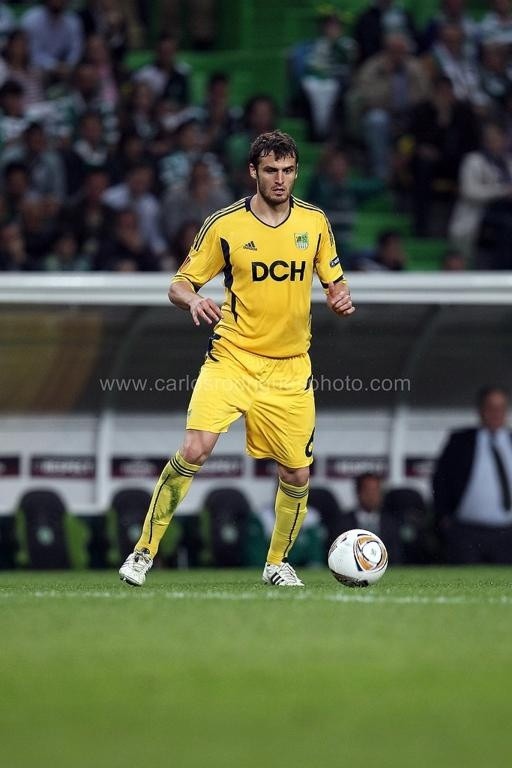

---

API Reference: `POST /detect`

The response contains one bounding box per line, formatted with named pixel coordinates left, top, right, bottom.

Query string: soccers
left=328, top=528, right=389, bottom=587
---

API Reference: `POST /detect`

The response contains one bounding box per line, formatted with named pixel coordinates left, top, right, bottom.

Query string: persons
left=335, top=469, right=401, bottom=556
left=119, top=127, right=354, bottom=588
left=430, top=384, right=510, bottom=568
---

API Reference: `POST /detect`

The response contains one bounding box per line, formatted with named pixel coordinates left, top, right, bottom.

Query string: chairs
left=1, top=484, right=430, bottom=572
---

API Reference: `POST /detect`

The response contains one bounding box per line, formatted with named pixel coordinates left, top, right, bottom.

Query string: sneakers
left=261, top=561, right=307, bottom=590
left=116, top=550, right=153, bottom=588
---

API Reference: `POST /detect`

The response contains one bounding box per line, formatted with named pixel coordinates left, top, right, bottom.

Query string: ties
left=488, top=440, right=510, bottom=511
